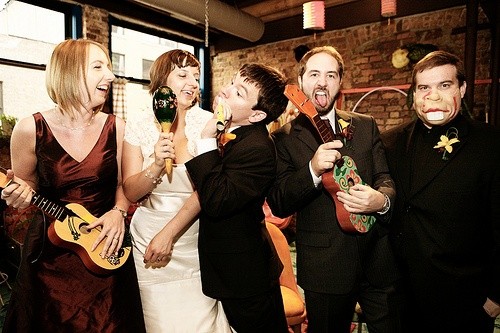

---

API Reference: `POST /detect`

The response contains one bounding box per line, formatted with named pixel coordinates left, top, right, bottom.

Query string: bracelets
left=145, top=167, right=161, bottom=184
left=112, top=206, right=128, bottom=219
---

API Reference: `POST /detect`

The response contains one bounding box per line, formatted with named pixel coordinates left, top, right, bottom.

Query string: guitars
left=285, top=84, right=377, bottom=234
left=0, top=167, right=132, bottom=273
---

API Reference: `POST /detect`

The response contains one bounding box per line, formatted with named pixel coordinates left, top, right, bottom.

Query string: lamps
left=303, top=1, right=325, bottom=40
left=380, top=0, right=397, bottom=25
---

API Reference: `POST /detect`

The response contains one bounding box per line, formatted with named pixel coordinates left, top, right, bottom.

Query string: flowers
left=337, top=119, right=351, bottom=146
left=433, top=127, right=460, bottom=161
left=218, top=132, right=236, bottom=157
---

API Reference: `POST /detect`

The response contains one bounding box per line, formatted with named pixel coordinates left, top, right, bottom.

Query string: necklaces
left=55, top=106, right=95, bottom=129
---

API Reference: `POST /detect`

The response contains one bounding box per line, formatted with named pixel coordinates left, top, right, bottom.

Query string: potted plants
left=0, top=114, right=18, bottom=136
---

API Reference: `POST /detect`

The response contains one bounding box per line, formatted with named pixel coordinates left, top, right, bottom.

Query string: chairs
left=265, top=221, right=307, bottom=333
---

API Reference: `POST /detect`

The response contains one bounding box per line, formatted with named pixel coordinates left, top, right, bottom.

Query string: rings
left=157, top=258, right=161, bottom=261
left=114, top=237, right=118, bottom=240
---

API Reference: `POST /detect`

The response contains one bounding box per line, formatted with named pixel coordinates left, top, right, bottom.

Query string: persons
left=121, top=49, right=237, bottom=333
left=381, top=50, right=500, bottom=333
left=265, top=46, right=397, bottom=333
left=184, top=64, right=289, bottom=333
left=1, top=39, right=133, bottom=333
